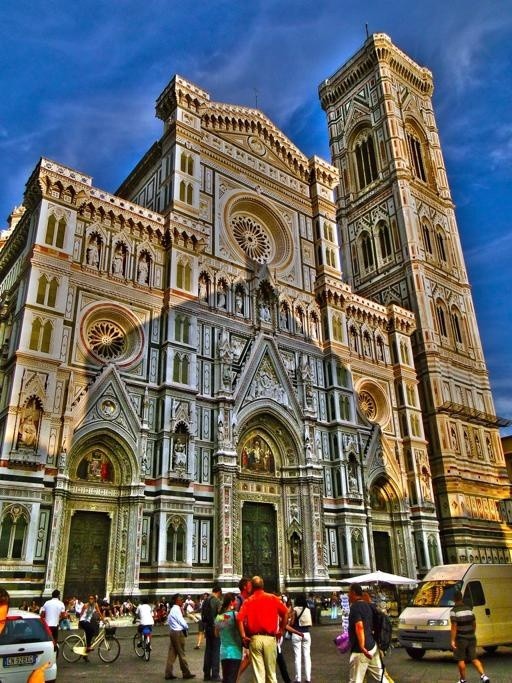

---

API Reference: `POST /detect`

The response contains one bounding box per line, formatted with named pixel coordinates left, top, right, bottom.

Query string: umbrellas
left=335, top=570, right=422, bottom=587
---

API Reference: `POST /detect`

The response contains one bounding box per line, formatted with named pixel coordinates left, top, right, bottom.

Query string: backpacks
left=371, top=605, right=391, bottom=650
left=202, top=596, right=214, bottom=623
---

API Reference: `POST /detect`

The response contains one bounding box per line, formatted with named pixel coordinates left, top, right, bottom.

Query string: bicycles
left=62, top=617, right=121, bottom=664
left=132, top=619, right=152, bottom=662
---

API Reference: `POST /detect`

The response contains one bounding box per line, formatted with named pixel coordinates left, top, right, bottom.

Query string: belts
left=252, top=632, right=275, bottom=637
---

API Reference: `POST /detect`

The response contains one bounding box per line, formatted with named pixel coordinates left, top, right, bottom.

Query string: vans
left=397, top=563, right=512, bottom=659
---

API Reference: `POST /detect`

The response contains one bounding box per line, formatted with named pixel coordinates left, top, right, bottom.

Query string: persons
left=249, top=367, right=289, bottom=407
left=241, top=441, right=276, bottom=473
left=172, top=438, right=187, bottom=466
left=447, top=592, right=490, bottom=682
left=258, top=295, right=383, bottom=361
left=235, top=290, right=244, bottom=313
left=216, top=284, right=226, bottom=308
left=1, top=577, right=395, bottom=682
left=137, top=256, right=148, bottom=284
left=113, top=249, right=124, bottom=278
left=198, top=277, right=208, bottom=301
left=87, top=240, right=100, bottom=266
left=77, top=455, right=115, bottom=481
left=18, top=414, right=36, bottom=446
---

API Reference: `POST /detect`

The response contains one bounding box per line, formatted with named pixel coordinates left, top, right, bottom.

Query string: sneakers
left=194, top=646, right=201, bottom=650
left=83, top=656, right=90, bottom=662
left=165, top=676, right=177, bottom=680
left=137, top=642, right=143, bottom=647
left=213, top=677, right=221, bottom=682
left=146, top=646, right=152, bottom=651
left=85, top=648, right=94, bottom=653
left=183, top=675, right=196, bottom=679
left=204, top=676, right=211, bottom=681
left=458, top=679, right=466, bottom=683
left=481, top=674, right=489, bottom=683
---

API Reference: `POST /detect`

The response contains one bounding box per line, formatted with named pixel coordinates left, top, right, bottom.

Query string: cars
left=0, top=609, right=59, bottom=683
